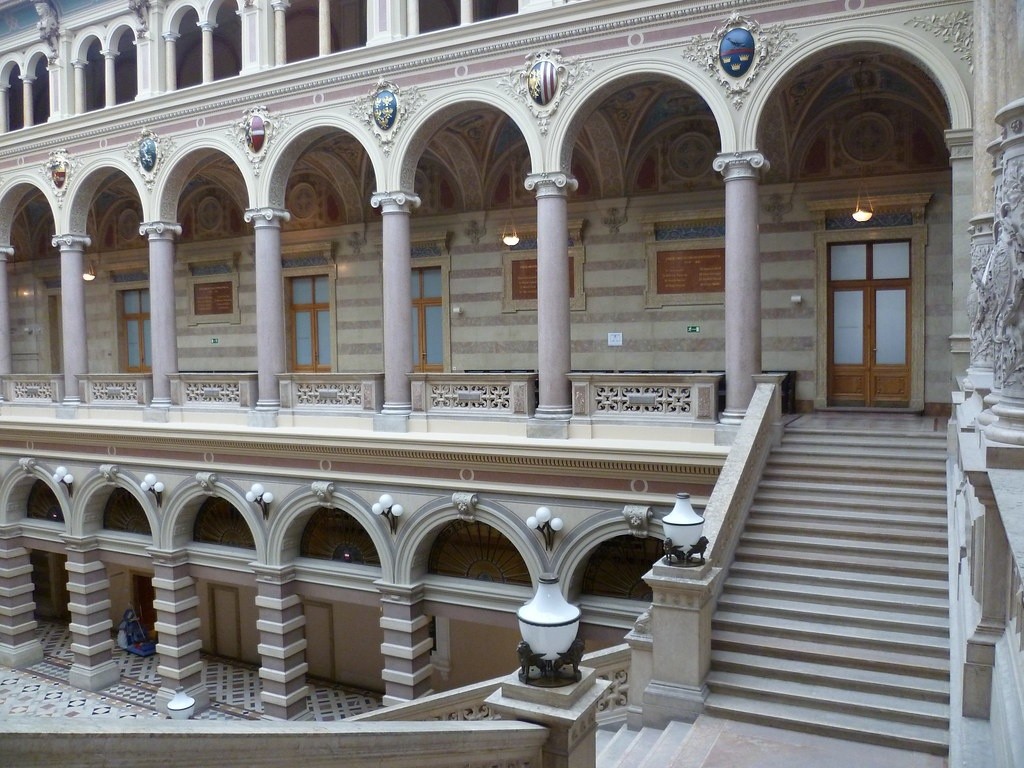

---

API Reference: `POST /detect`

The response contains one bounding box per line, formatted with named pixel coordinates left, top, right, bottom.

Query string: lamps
left=499, top=153, right=519, bottom=246
left=246, top=482, right=273, bottom=520
left=851, top=55, right=874, bottom=222
left=527, top=507, right=563, bottom=552
left=53, top=465, right=73, bottom=497
left=140, top=473, right=165, bottom=507
left=372, top=494, right=403, bottom=534
left=661, top=493, right=708, bottom=568
left=82, top=259, right=97, bottom=282
left=516, top=574, right=586, bottom=687
left=167, top=684, right=195, bottom=719
